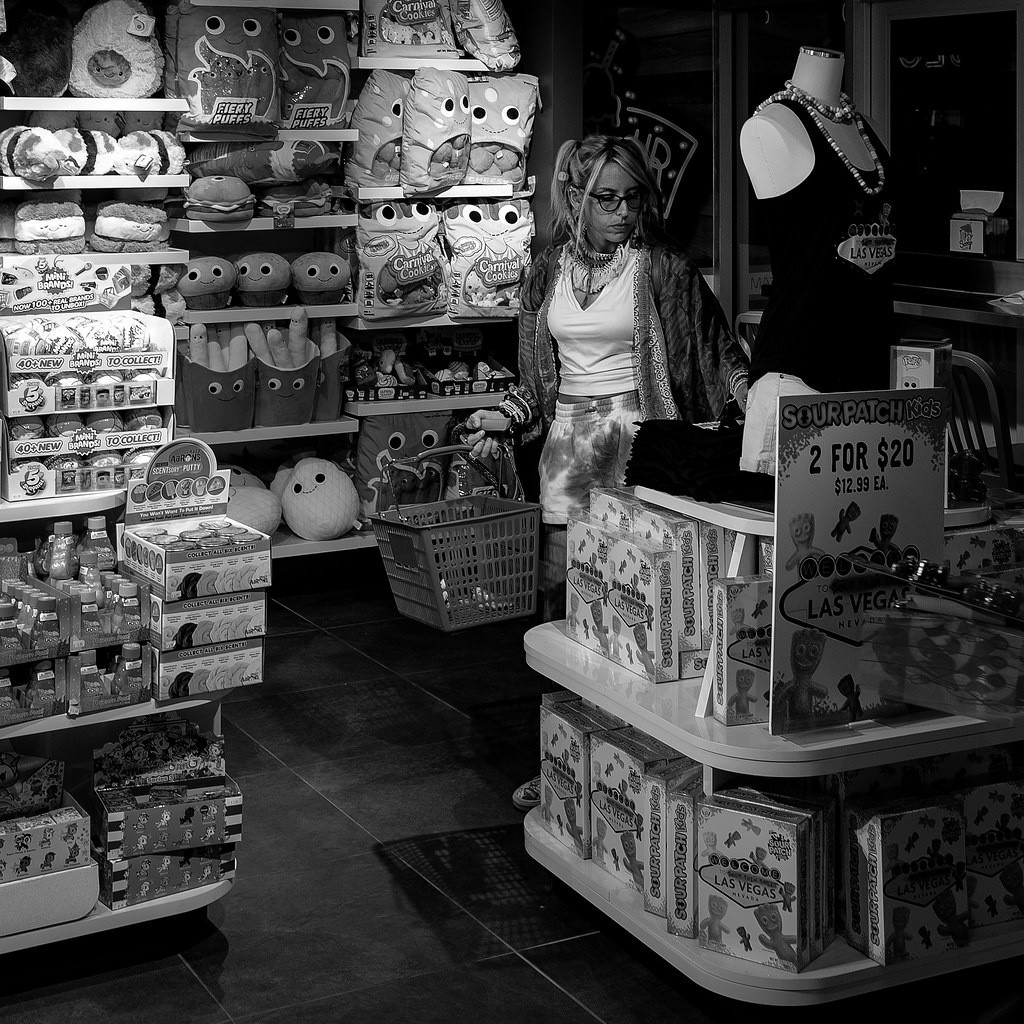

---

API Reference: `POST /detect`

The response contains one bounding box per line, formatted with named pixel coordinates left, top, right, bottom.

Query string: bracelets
left=492, top=382, right=539, bottom=432
left=726, top=367, right=749, bottom=404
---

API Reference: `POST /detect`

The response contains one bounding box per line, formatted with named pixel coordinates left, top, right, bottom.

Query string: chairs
left=946, top=350, right=1024, bottom=495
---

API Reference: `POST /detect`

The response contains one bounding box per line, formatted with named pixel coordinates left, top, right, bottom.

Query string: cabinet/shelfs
left=0, top=0, right=514, bottom=559
left=523, top=486, right=1024, bottom=1005
left=0, top=488, right=238, bottom=954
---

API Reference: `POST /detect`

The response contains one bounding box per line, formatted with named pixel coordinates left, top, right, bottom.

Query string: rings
left=469, top=452, right=478, bottom=460
left=465, top=436, right=469, bottom=445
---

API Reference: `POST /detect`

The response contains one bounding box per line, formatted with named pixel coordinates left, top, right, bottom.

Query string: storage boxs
left=541, top=486, right=1024, bottom=975
left=421, top=356, right=517, bottom=396
left=0, top=310, right=272, bottom=936
left=343, top=368, right=428, bottom=403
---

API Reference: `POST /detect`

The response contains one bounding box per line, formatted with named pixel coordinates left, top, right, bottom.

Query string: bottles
left=0, top=515, right=142, bottom=711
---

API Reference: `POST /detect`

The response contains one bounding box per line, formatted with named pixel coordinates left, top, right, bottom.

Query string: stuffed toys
left=351, top=0, right=540, bottom=517
left=132, top=8, right=362, bottom=546
left=0, top=0, right=185, bottom=255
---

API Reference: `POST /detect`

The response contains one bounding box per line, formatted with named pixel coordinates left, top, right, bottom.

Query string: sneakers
left=511, top=774, right=541, bottom=807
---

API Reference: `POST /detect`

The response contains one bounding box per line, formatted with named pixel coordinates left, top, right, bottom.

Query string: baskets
left=368, top=443, right=545, bottom=634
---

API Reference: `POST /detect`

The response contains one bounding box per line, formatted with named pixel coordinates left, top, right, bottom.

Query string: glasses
left=577, top=188, right=648, bottom=212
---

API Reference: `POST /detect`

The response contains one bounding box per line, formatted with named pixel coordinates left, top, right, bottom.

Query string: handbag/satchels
left=444, top=424, right=525, bottom=502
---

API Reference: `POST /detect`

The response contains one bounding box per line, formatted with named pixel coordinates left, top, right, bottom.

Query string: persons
left=465, top=135, right=747, bottom=811
left=739, top=46, right=900, bottom=503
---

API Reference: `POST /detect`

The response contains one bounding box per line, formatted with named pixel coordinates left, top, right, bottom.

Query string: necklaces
left=559, top=221, right=631, bottom=294
left=752, top=80, right=885, bottom=195
